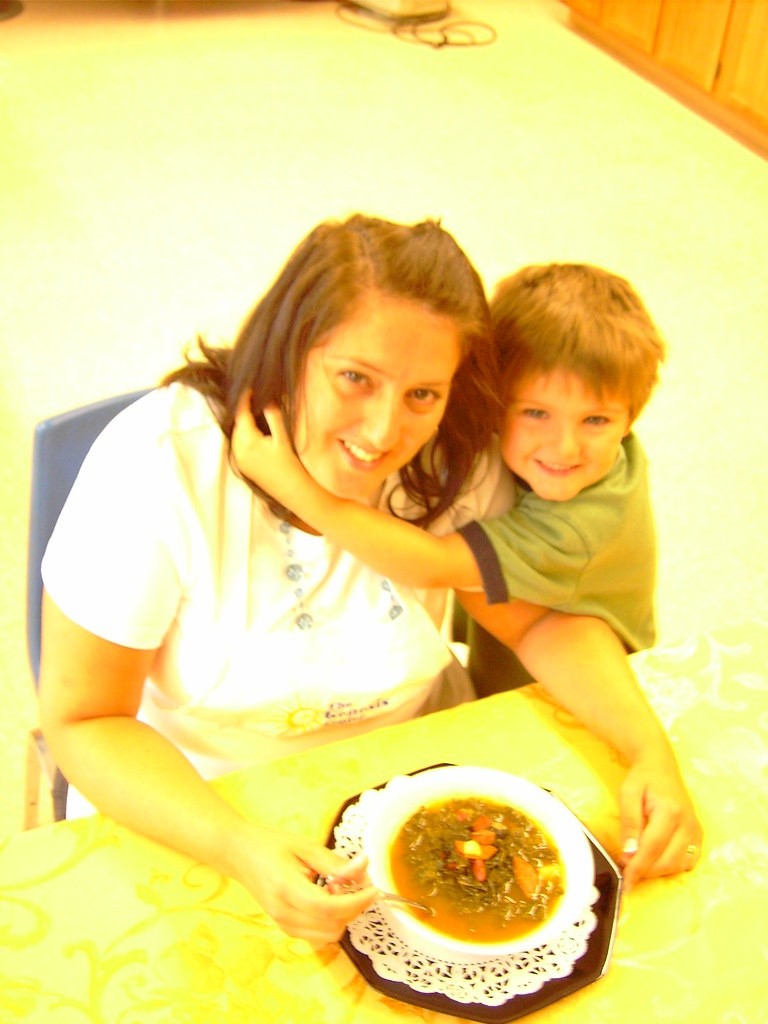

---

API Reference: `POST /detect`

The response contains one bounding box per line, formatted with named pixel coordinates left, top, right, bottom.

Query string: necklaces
left=281, top=508, right=405, bottom=634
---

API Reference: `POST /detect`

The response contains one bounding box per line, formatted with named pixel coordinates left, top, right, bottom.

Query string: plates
left=363, top=765, right=595, bottom=955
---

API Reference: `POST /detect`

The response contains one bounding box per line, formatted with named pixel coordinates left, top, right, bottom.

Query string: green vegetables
left=405, top=810, right=518, bottom=907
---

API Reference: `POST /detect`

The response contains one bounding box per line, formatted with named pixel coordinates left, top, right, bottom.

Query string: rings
left=685, top=845, right=696, bottom=856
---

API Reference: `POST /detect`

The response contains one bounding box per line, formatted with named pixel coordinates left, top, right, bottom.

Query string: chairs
left=22, top=387, right=158, bottom=832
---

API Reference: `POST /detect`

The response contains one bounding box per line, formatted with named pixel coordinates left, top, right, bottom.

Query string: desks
left=0, top=624, right=768, bottom=1022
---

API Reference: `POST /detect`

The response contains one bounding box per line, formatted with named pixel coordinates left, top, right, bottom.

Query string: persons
left=229, top=261, right=665, bottom=700
left=38, top=214, right=704, bottom=948
